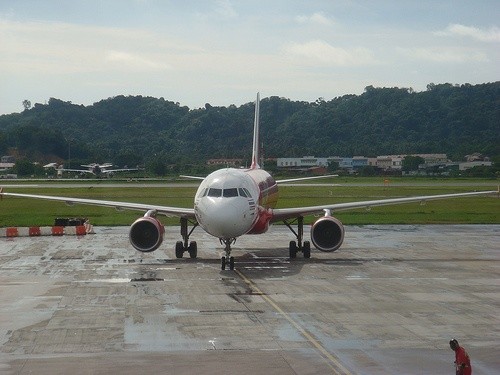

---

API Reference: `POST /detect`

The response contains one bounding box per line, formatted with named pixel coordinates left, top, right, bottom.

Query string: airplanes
left=0, top=92, right=499, bottom=272
left=55, top=161, right=146, bottom=179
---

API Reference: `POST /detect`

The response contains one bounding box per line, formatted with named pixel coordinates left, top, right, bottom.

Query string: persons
left=450, top=338, right=471, bottom=375
